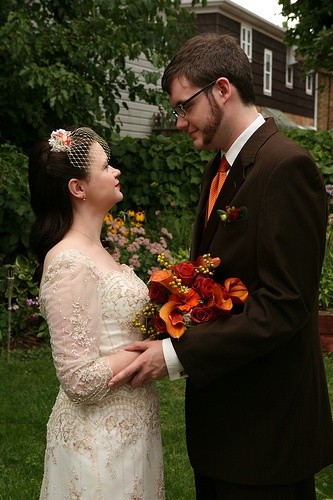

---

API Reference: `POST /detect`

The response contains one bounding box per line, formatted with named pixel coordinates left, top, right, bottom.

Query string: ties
left=208, top=154, right=229, bottom=221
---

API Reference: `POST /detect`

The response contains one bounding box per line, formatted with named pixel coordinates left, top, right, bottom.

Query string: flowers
left=128, top=253, right=248, bottom=339
left=216, top=204, right=239, bottom=221
left=48, top=128, right=74, bottom=152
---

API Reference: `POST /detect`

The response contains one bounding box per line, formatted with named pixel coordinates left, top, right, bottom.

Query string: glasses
left=172, top=81, right=215, bottom=118
